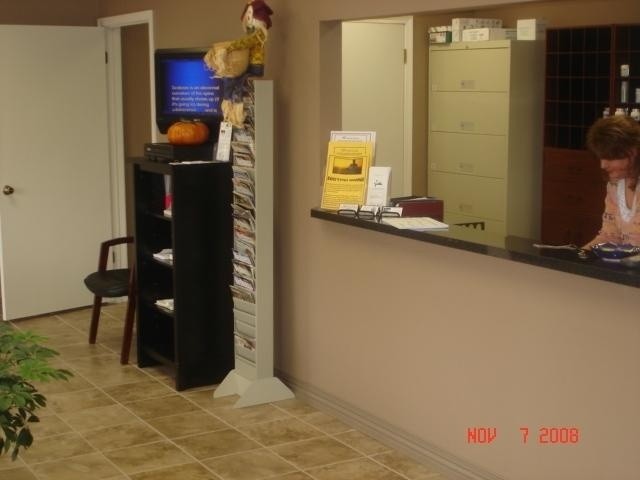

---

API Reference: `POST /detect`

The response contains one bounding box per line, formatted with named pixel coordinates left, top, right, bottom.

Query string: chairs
left=84, top=235, right=135, bottom=365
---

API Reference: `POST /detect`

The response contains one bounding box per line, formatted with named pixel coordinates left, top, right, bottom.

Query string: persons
left=577, top=116, right=640, bottom=262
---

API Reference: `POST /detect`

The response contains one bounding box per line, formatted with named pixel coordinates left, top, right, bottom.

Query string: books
left=215, top=115, right=255, bottom=355
left=319, top=128, right=394, bottom=211
left=382, top=215, right=450, bottom=233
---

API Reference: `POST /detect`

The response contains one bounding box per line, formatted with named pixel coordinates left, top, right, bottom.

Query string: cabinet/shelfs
left=615, top=22, right=640, bottom=119
left=132, top=154, right=234, bottom=391
left=543, top=24, right=616, bottom=245
left=425, top=42, right=546, bottom=247
left=212, top=80, right=293, bottom=405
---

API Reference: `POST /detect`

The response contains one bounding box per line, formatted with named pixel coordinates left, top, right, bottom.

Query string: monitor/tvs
left=154, top=48, right=224, bottom=143
